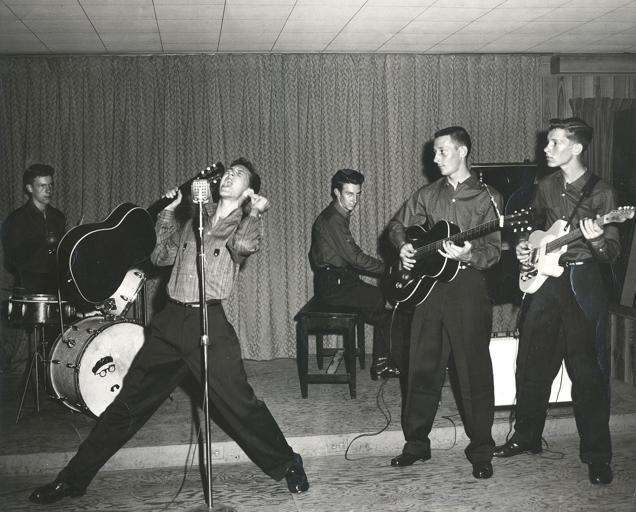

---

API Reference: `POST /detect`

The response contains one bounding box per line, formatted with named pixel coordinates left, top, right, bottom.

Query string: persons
left=309, top=168, right=402, bottom=381
left=387, top=125, right=503, bottom=480
left=492, top=117, right=622, bottom=483
left=1, top=163, right=67, bottom=338
left=29, top=157, right=309, bottom=506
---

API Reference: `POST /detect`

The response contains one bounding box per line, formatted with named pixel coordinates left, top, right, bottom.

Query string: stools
left=294, top=296, right=366, bottom=398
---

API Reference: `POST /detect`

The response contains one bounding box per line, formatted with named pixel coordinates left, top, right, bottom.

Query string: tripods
left=15, top=325, right=48, bottom=427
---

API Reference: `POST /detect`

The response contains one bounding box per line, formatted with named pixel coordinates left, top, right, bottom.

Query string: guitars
left=384, top=206, right=536, bottom=307
left=516, top=206, right=635, bottom=293
left=56, top=162, right=225, bottom=313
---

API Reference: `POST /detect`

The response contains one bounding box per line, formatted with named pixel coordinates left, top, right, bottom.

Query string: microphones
left=190, top=180, right=211, bottom=204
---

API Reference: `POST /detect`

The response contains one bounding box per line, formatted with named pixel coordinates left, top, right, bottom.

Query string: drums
left=7, top=293, right=76, bottom=329
left=47, top=315, right=146, bottom=419
left=94, top=269, right=148, bottom=316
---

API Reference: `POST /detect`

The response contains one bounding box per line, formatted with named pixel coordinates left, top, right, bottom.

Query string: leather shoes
left=491, top=440, right=542, bottom=458
left=286, top=465, right=308, bottom=493
left=588, top=463, right=612, bottom=484
left=473, top=464, right=492, bottom=479
left=370, top=365, right=400, bottom=381
left=390, top=452, right=430, bottom=468
left=28, top=480, right=85, bottom=505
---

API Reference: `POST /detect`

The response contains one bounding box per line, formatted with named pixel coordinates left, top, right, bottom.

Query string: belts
left=167, top=297, right=220, bottom=309
